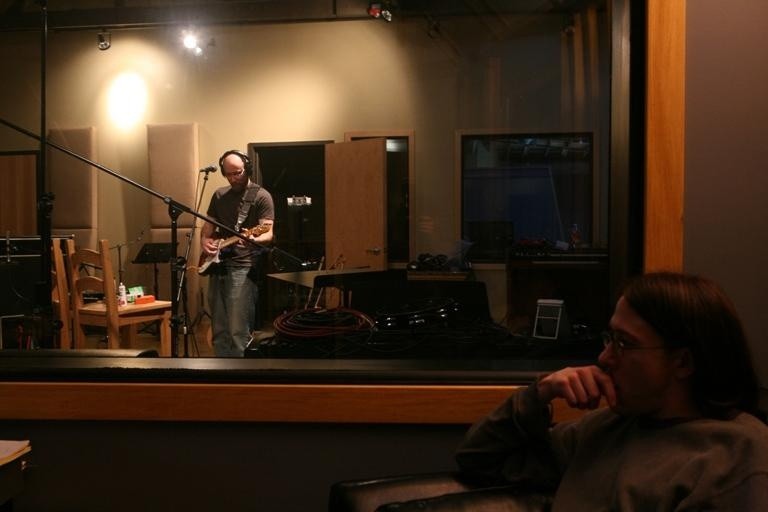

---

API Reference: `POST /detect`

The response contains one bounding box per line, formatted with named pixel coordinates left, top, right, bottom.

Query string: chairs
left=48, top=237, right=174, bottom=358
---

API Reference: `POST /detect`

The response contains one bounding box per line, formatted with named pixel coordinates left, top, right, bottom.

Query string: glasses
left=601, top=330, right=677, bottom=359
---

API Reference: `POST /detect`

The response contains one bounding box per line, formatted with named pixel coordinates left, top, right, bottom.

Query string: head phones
left=219, top=150, right=253, bottom=177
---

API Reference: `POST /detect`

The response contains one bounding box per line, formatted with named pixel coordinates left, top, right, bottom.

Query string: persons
left=198, top=150, right=276, bottom=358
left=455, top=270, right=766, bottom=509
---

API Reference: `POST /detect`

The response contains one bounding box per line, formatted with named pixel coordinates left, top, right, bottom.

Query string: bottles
left=118, top=282, right=128, bottom=307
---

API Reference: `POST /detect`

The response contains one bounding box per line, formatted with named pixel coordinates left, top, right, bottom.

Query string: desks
left=266, top=262, right=607, bottom=347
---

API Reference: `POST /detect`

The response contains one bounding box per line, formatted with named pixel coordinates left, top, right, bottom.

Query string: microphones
left=199, top=165, right=217, bottom=173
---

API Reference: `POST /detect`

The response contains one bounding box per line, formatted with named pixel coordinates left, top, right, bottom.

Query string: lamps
left=98, top=32, right=110, bottom=50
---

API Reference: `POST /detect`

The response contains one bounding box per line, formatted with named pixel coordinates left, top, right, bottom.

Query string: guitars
left=198, top=221, right=271, bottom=277
